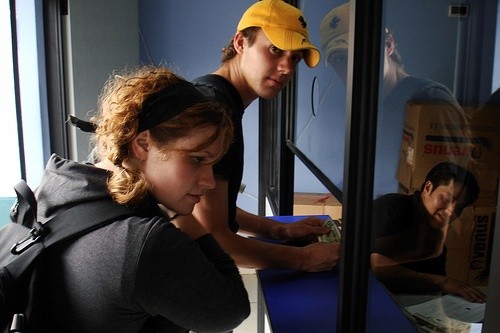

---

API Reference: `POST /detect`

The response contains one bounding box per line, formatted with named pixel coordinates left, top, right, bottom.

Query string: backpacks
left=2, top=180, right=170, bottom=333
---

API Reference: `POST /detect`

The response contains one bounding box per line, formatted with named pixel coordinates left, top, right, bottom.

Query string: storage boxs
left=395, top=99, right=500, bottom=287
left=293, top=192, right=342, bottom=220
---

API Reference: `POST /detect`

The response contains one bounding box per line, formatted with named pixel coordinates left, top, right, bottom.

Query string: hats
left=321, top=2, right=350, bottom=67
left=237, top=0, right=320, bottom=69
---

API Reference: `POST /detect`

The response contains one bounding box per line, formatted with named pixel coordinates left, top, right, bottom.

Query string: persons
left=13, top=67, right=251, bottom=333
left=189, top=0, right=340, bottom=273
left=369, top=161, right=488, bottom=303
left=321, top=2, right=464, bottom=194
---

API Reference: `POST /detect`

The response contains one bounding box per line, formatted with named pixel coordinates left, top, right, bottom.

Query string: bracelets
left=171, top=214, right=178, bottom=221
